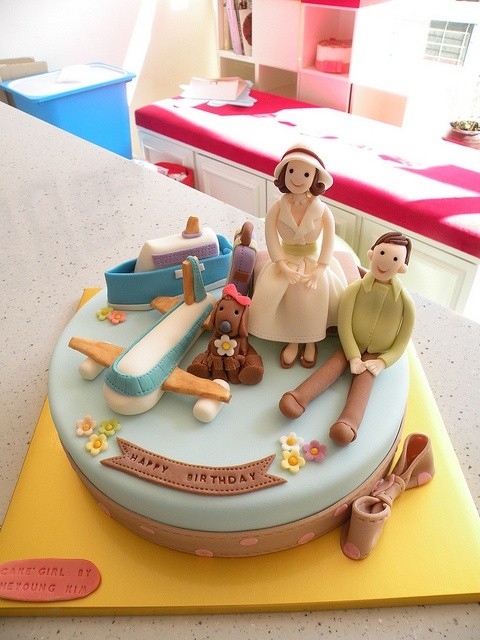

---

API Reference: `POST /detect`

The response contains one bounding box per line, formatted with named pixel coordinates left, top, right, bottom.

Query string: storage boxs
left=315, top=41, right=351, bottom=72
left=2, top=62, right=136, bottom=158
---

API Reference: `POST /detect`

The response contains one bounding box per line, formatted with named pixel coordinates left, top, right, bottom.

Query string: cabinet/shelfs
left=213, top=0, right=425, bottom=126
left=266, top=180, right=358, bottom=271
left=358, top=217, right=478, bottom=313
left=137, top=130, right=266, bottom=226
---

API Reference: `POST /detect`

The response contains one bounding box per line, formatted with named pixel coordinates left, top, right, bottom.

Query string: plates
left=451, top=127, right=480, bottom=136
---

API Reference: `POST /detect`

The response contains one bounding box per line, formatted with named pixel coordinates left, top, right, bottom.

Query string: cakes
left=45, top=141, right=436, bottom=561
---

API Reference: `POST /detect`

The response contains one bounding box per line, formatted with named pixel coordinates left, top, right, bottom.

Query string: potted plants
left=445, top=120, right=479, bottom=150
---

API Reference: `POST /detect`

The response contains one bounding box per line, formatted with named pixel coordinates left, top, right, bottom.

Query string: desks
left=0, top=102, right=480, bottom=639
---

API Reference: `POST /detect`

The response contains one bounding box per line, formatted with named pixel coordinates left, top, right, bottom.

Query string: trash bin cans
left=155, top=162, right=194, bottom=188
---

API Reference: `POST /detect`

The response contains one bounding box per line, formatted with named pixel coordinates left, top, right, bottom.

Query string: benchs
left=134, top=84, right=480, bottom=315
